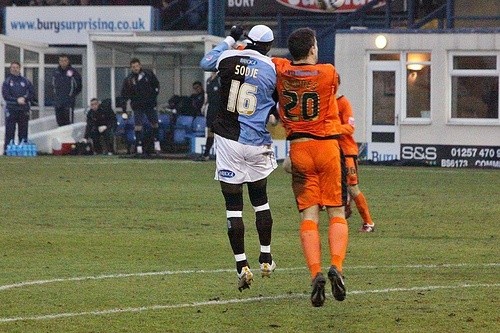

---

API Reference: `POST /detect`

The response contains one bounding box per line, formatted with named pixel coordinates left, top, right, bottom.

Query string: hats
left=242, top=25, right=274, bottom=43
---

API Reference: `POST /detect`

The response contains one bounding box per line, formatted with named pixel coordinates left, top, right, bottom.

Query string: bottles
left=6, top=144, right=36, bottom=157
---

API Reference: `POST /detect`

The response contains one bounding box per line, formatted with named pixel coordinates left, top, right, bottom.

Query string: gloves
left=230, top=23, right=244, bottom=40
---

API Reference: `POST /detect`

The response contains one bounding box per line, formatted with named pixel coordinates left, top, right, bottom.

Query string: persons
left=115, top=57, right=164, bottom=161
left=52, top=53, right=82, bottom=127
left=2, top=62, right=35, bottom=156
left=232, top=28, right=375, bottom=307
left=79, top=98, right=117, bottom=156
left=199, top=25, right=276, bottom=292
left=159, top=81, right=205, bottom=116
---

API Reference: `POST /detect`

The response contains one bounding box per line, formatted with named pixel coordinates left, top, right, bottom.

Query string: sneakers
left=327, top=265, right=346, bottom=301
left=236, top=266, right=254, bottom=293
left=259, top=260, right=276, bottom=278
left=310, top=273, right=326, bottom=307
left=358, top=222, right=375, bottom=234
left=344, top=205, right=353, bottom=219
left=194, top=154, right=209, bottom=162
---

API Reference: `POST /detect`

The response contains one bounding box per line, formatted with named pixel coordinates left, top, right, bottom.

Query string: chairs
left=112, top=111, right=206, bottom=155
